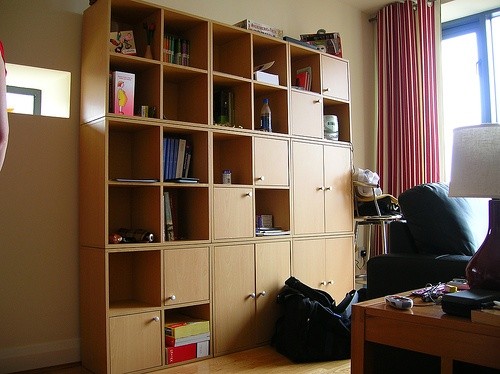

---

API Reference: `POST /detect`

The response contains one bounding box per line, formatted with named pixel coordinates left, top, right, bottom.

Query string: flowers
left=144, top=21, right=156, bottom=44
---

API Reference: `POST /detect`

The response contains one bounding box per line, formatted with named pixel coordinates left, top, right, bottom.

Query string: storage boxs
left=232, top=19, right=283, bottom=39
left=111, top=71, right=136, bottom=116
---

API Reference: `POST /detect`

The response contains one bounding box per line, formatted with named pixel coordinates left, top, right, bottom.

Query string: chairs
left=352, top=180, right=403, bottom=283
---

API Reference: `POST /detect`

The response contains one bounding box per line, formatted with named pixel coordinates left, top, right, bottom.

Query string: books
left=163, top=191, right=178, bottom=242
left=113, top=178, right=160, bottom=183
left=163, top=138, right=200, bottom=183
left=163, top=34, right=191, bottom=67
left=256, top=227, right=291, bottom=236
left=295, top=66, right=312, bottom=91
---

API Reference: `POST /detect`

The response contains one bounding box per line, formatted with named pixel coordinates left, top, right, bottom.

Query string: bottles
left=259, top=98, right=273, bottom=132
left=222, top=170, right=232, bottom=184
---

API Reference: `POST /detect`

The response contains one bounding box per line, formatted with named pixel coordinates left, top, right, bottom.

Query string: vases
left=144, top=45, right=154, bottom=59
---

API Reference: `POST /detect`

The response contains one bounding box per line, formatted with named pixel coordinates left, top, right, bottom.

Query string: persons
left=0, top=39, right=9, bottom=172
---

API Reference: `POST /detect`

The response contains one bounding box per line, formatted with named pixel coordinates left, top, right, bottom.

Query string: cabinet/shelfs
left=79, top=0, right=357, bottom=374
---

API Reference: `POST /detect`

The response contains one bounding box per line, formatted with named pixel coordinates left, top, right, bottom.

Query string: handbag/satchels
left=352, top=169, right=382, bottom=199
left=270, top=277, right=359, bottom=364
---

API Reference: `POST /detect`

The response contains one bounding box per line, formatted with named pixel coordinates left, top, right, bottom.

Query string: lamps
left=447, top=123, right=500, bottom=293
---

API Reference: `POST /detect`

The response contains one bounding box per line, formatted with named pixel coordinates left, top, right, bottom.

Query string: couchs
left=359, top=181, right=500, bottom=374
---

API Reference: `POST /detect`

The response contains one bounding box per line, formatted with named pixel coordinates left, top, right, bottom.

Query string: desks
left=351, top=288, right=500, bottom=374
